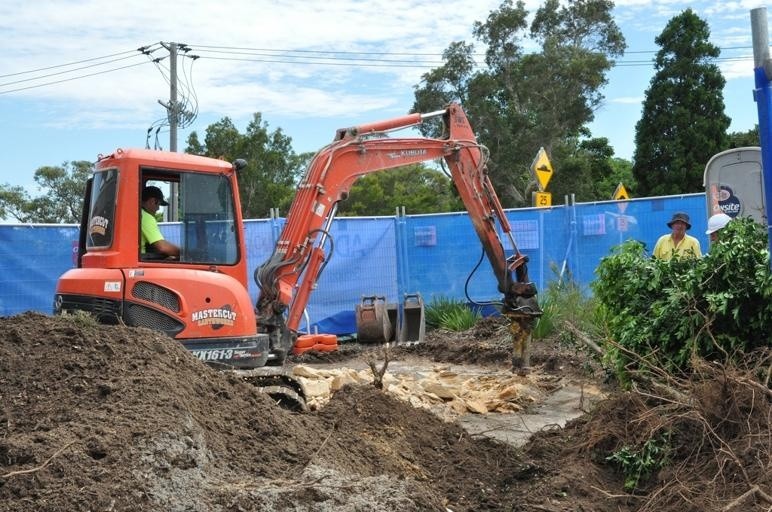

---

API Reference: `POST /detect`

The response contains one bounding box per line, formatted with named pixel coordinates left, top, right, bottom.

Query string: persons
left=652, top=213, right=702, bottom=267
left=705, top=214, right=732, bottom=243
left=138, top=185, right=181, bottom=260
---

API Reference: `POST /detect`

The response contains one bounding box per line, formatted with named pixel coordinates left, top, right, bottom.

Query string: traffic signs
left=534, top=190, right=552, bottom=208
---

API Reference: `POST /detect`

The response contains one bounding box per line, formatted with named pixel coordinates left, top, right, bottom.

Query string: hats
left=667, top=214, right=691, bottom=230
left=705, top=214, right=730, bottom=234
left=143, top=187, right=170, bottom=206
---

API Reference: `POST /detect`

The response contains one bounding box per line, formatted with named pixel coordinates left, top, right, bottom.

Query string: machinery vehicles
left=50, top=98, right=568, bottom=414
